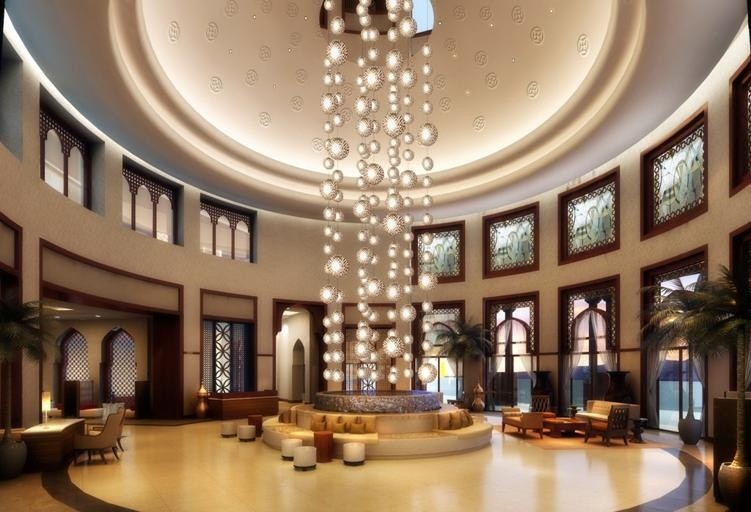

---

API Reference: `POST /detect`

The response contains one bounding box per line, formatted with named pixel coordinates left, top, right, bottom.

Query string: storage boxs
left=502, top=407, right=545, bottom=439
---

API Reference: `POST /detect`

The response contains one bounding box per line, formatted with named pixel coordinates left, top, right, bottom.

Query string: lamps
left=89, top=405, right=126, bottom=461
left=74, top=410, right=119, bottom=465
left=530, top=394, right=557, bottom=433
left=584, top=405, right=630, bottom=446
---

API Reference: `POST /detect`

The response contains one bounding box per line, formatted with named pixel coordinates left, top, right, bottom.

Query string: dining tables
left=434, top=315, right=496, bottom=407
left=634, top=274, right=705, bottom=445
left=1, top=295, right=59, bottom=479
left=642, top=263, right=751, bottom=512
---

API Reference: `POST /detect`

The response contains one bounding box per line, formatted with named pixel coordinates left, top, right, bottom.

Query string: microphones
left=629, top=417, right=648, bottom=444
left=221, top=413, right=369, bottom=470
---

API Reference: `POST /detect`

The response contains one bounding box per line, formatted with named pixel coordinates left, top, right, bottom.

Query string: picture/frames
left=21, top=417, right=85, bottom=465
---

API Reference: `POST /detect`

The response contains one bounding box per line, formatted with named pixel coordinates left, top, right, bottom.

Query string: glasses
left=320, top=1, right=439, bottom=389
left=42, top=391, right=52, bottom=428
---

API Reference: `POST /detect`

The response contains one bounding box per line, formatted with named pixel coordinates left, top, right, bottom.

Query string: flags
left=532, top=370, right=555, bottom=411
left=605, top=370, right=633, bottom=402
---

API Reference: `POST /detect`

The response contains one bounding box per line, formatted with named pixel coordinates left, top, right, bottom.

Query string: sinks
left=575, top=399, right=641, bottom=445
